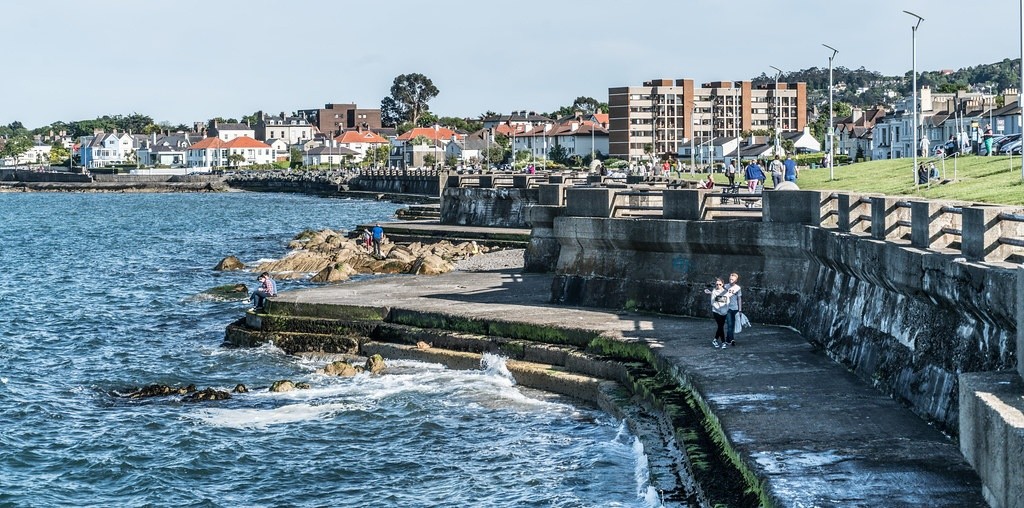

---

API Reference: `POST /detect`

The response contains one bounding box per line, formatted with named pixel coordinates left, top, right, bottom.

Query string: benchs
left=741, top=185, right=764, bottom=208
left=702, top=181, right=715, bottom=188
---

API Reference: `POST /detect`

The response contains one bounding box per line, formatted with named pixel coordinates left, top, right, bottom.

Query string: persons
left=362, top=228, right=372, bottom=245
left=700, top=174, right=712, bottom=189
left=783, top=157, right=798, bottom=183
left=820, top=152, right=830, bottom=168
left=242, top=272, right=277, bottom=313
left=963, top=124, right=993, bottom=156
left=599, top=163, right=607, bottom=182
left=704, top=273, right=742, bottom=345
left=711, top=277, right=730, bottom=349
left=920, top=135, right=930, bottom=157
left=524, top=164, right=534, bottom=174
left=769, top=155, right=783, bottom=187
left=629, top=158, right=685, bottom=178
left=745, top=159, right=767, bottom=193
left=728, top=160, right=736, bottom=185
left=918, top=161, right=938, bottom=183
left=371, top=222, right=383, bottom=254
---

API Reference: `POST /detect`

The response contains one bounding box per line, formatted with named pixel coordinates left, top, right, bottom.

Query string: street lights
left=822, top=43, right=839, bottom=182
left=903, top=9, right=925, bottom=186
left=985, top=83, right=995, bottom=133
left=368, top=132, right=396, bottom=170
left=133, top=136, right=143, bottom=163
left=731, top=80, right=742, bottom=178
left=426, top=123, right=441, bottom=170
left=708, top=93, right=717, bottom=174
left=769, top=65, right=783, bottom=154
left=689, top=101, right=697, bottom=173
left=482, top=123, right=547, bottom=175
left=587, top=120, right=596, bottom=161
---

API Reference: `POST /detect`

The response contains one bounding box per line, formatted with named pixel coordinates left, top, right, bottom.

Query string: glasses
left=716, top=284, right=722, bottom=286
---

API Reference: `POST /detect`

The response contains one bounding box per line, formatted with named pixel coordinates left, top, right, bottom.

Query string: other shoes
left=727, top=342, right=732, bottom=345
left=731, top=340, right=736, bottom=346
left=722, top=342, right=728, bottom=349
left=249, top=308, right=256, bottom=311
left=712, top=340, right=720, bottom=348
left=242, top=300, right=250, bottom=304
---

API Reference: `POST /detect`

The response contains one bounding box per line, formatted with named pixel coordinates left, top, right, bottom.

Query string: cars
left=963, top=133, right=1023, bottom=155
left=500, top=164, right=512, bottom=170
